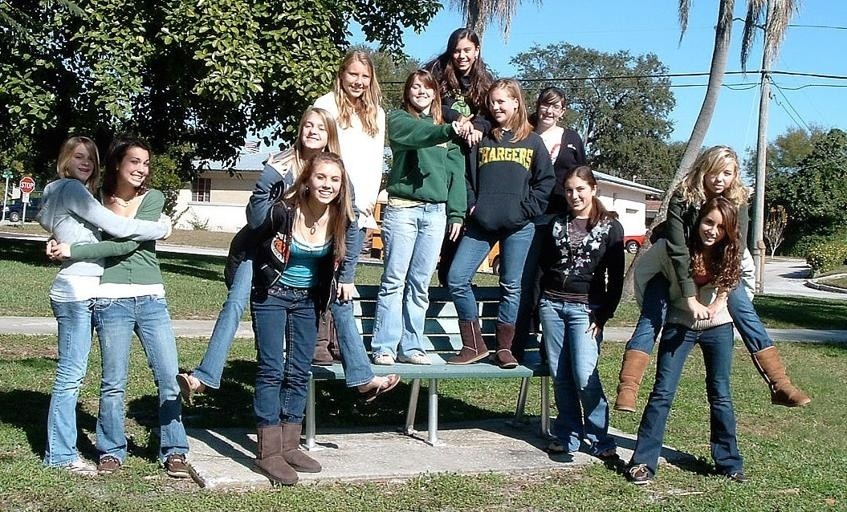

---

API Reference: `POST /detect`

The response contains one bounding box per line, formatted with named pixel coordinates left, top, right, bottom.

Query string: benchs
left=301, top=281, right=558, bottom=453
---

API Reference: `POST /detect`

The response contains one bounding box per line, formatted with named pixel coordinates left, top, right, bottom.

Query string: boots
left=282, top=422, right=322, bottom=473
left=313, top=310, right=333, bottom=366
left=447, top=319, right=489, bottom=365
left=251, top=425, right=299, bottom=485
left=494, top=323, right=518, bottom=368
left=614, top=349, right=650, bottom=413
left=751, top=346, right=812, bottom=407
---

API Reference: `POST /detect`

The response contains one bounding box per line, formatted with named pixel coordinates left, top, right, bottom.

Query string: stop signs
left=19, top=176, right=36, bottom=194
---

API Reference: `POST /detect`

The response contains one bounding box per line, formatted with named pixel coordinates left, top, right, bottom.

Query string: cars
left=623, top=230, right=648, bottom=253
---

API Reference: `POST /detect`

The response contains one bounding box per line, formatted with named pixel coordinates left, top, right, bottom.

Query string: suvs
left=0, top=197, right=42, bottom=222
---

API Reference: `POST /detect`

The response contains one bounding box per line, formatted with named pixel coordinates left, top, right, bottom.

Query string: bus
left=370, top=187, right=501, bottom=278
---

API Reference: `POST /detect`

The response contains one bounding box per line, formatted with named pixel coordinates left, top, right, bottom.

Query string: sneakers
left=549, top=440, right=579, bottom=451
left=731, top=473, right=747, bottom=482
left=629, top=463, right=649, bottom=485
left=167, top=454, right=190, bottom=478
left=62, top=458, right=98, bottom=476
left=397, top=353, right=432, bottom=365
left=98, top=456, right=121, bottom=475
left=373, top=353, right=395, bottom=365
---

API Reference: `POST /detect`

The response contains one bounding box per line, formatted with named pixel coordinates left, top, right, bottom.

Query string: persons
left=36, top=136, right=173, bottom=473
left=177, top=107, right=400, bottom=406
left=615, top=145, right=811, bottom=413
left=311, top=47, right=384, bottom=363
left=511, top=86, right=587, bottom=364
left=370, top=69, right=472, bottom=365
left=46, top=140, right=188, bottom=478
left=223, top=153, right=354, bottom=486
left=445, top=79, right=557, bottom=367
left=426, top=29, right=494, bottom=286
left=628, top=198, right=756, bottom=485
left=538, top=167, right=622, bottom=457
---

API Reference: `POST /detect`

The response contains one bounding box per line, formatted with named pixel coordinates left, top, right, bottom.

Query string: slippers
left=176, top=374, right=200, bottom=408
left=366, top=374, right=400, bottom=403
